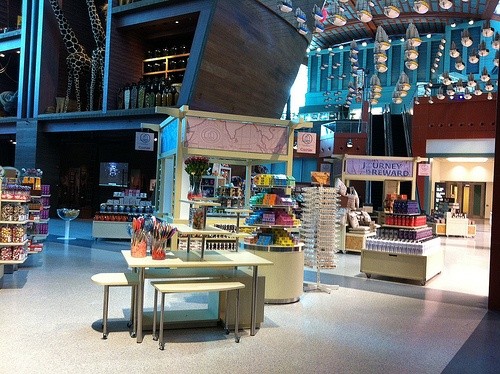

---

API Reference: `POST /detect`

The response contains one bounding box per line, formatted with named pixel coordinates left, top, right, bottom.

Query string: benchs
left=90, top=272, right=137, bottom=340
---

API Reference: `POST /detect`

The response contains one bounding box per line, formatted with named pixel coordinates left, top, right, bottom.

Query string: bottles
left=0, top=224, right=24, bottom=244
left=0, top=245, right=25, bottom=261
left=1, top=203, right=29, bottom=221
left=365, top=213, right=469, bottom=255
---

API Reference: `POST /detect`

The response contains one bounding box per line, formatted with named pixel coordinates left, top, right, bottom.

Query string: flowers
left=184, top=154, right=209, bottom=174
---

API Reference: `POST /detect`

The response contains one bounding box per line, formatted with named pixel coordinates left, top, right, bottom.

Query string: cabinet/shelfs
left=300, top=187, right=341, bottom=294
left=382, top=210, right=427, bottom=230
left=142, top=52, right=190, bottom=79
left=240, top=184, right=302, bottom=228
left=202, top=164, right=231, bottom=197
left=0, top=195, right=51, bottom=264
left=100, top=203, right=133, bottom=214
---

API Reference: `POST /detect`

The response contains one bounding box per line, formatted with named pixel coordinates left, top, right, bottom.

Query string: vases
left=187, top=175, right=203, bottom=200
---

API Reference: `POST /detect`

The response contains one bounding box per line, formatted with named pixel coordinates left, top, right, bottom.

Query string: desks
left=445, top=211, right=468, bottom=238
left=120, top=250, right=274, bottom=339
left=169, top=223, right=232, bottom=261
left=178, top=200, right=221, bottom=228
left=427, top=222, right=446, bottom=237
left=468, top=225, right=476, bottom=238
left=92, top=221, right=132, bottom=241
left=150, top=279, right=246, bottom=351
left=346, top=233, right=372, bottom=253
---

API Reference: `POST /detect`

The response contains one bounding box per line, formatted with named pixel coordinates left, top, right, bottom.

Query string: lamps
left=278, top=0, right=500, bottom=108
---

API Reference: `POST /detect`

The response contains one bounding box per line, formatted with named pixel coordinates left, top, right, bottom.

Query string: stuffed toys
left=85, top=0, right=106, bottom=111
left=49, top=0, right=92, bottom=113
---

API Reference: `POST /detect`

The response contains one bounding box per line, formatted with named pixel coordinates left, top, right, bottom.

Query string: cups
left=151, top=240, right=167, bottom=260
left=130, top=235, right=147, bottom=258
